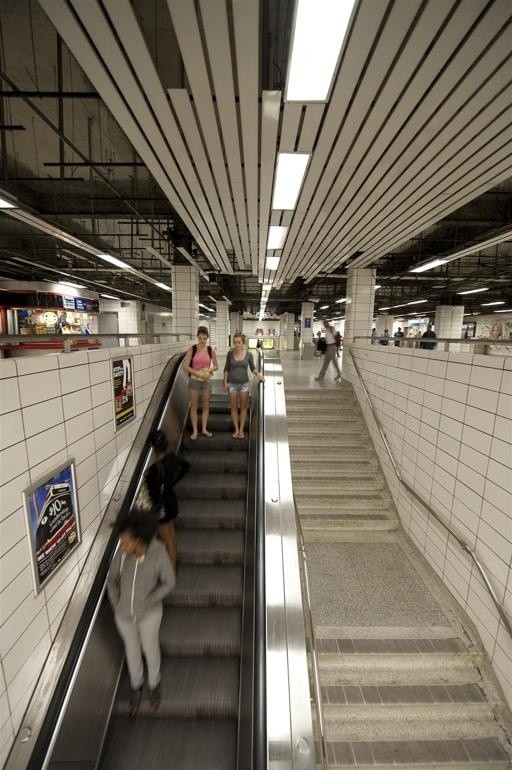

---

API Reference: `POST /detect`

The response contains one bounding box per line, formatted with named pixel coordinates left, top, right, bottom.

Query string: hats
left=323, top=318, right=332, bottom=324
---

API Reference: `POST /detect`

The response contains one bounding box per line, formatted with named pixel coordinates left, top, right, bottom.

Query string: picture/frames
left=21, top=458, right=83, bottom=597
left=109, top=355, right=137, bottom=432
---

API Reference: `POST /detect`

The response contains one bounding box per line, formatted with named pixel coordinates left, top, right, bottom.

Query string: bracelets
left=253, top=370, right=258, bottom=375
left=213, top=367, right=218, bottom=371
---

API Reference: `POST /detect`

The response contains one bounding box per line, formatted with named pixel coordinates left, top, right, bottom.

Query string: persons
left=182, top=325, right=219, bottom=439
left=293, top=327, right=421, bottom=358
left=491, top=322, right=502, bottom=340
left=222, top=333, right=266, bottom=439
left=144, top=429, right=191, bottom=570
left=106, top=510, right=176, bottom=721
left=315, top=320, right=342, bottom=381
left=54, top=315, right=71, bottom=334
left=420, top=324, right=437, bottom=350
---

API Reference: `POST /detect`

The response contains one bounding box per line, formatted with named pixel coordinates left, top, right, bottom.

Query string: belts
left=326, top=343, right=338, bottom=346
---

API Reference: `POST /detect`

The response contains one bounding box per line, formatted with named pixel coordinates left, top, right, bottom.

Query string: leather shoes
left=128, top=689, right=143, bottom=721
left=334, top=375, right=342, bottom=381
left=315, top=377, right=322, bottom=381
left=146, top=684, right=163, bottom=715
